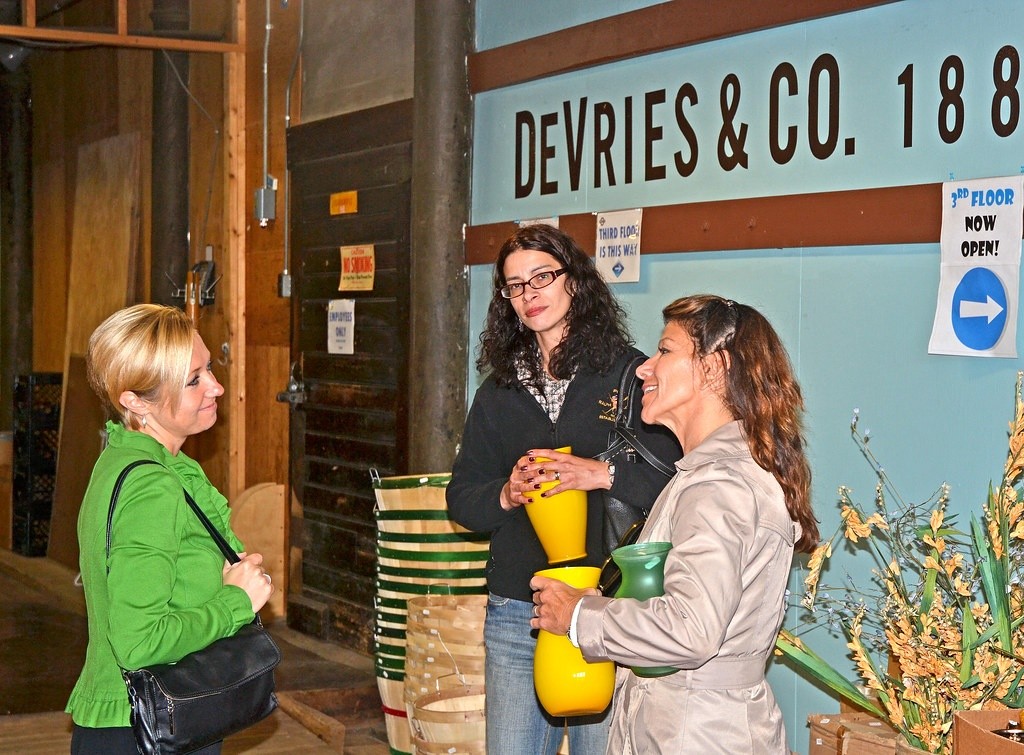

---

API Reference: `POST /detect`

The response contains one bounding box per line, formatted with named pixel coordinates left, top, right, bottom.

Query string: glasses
left=500, top=265, right=572, bottom=299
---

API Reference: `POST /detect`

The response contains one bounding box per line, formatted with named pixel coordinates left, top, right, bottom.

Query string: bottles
left=610, top=541, right=680, bottom=678
left=523, top=447, right=589, bottom=564
left=532, top=567, right=615, bottom=718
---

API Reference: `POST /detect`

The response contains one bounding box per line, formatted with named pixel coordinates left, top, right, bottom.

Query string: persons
left=444, top=224, right=685, bottom=754
left=64, top=301, right=276, bottom=755
left=530, top=295, right=823, bottom=755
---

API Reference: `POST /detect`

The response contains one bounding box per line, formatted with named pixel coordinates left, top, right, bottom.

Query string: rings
left=262, top=572, right=272, bottom=585
left=533, top=605, right=539, bottom=617
left=554, top=471, right=560, bottom=481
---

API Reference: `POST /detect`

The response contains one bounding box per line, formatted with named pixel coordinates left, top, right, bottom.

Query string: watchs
left=607, top=460, right=615, bottom=485
left=565, top=624, right=571, bottom=642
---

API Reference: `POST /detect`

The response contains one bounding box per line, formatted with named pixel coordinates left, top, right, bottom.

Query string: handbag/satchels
left=123, top=622, right=282, bottom=755
left=592, top=355, right=651, bottom=558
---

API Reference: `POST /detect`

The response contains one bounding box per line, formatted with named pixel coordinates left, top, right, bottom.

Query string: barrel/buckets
left=366, top=465, right=488, bottom=755
